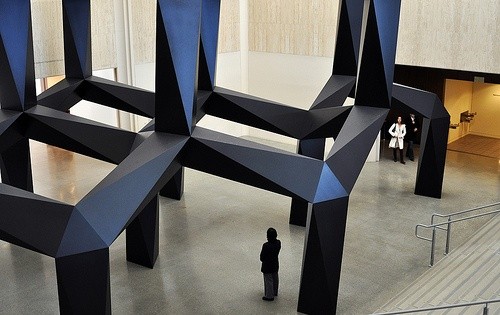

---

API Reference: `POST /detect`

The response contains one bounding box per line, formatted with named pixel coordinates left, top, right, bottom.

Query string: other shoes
left=394, top=159, right=397, bottom=162
left=400, top=160, right=405, bottom=164
left=262, top=297, right=274, bottom=301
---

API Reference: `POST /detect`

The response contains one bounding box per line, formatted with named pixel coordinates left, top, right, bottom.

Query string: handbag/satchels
left=388, top=123, right=396, bottom=138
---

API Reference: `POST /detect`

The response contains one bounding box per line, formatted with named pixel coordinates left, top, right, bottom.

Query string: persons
left=260, top=227, right=281, bottom=302
left=407, top=114, right=417, bottom=162
left=388, top=117, right=406, bottom=164
